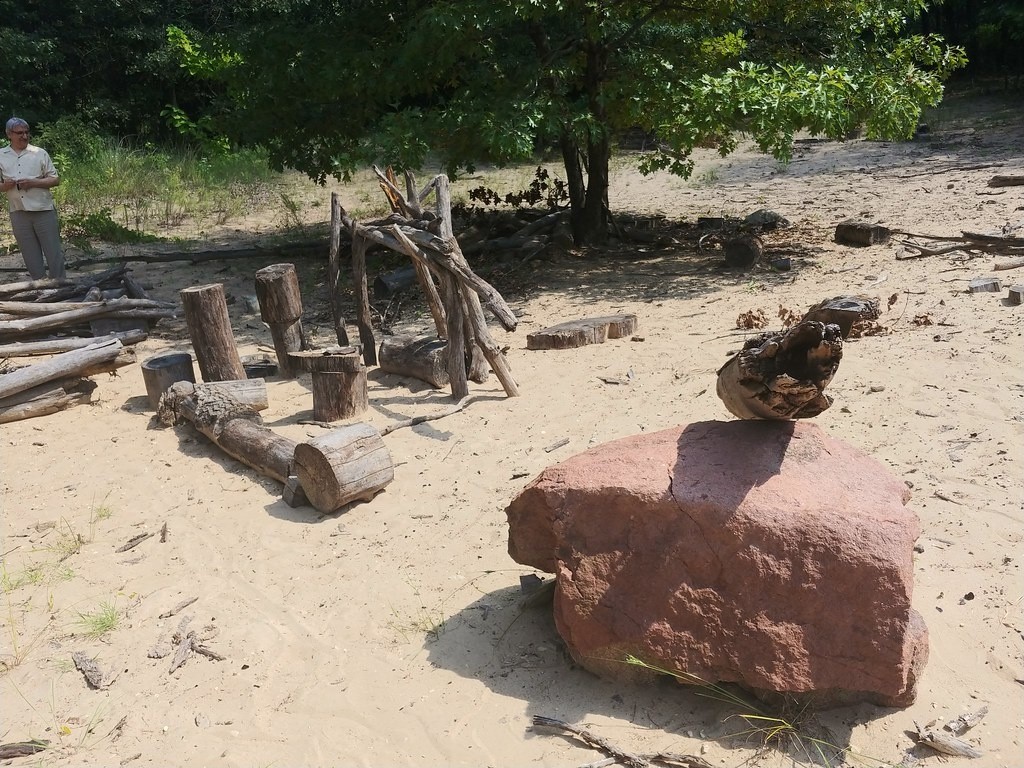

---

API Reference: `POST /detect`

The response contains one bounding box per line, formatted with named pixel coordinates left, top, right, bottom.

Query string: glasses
left=10, top=130, right=30, bottom=135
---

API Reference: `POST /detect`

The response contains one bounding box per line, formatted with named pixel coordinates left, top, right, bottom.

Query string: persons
left=0, top=118, right=67, bottom=280
